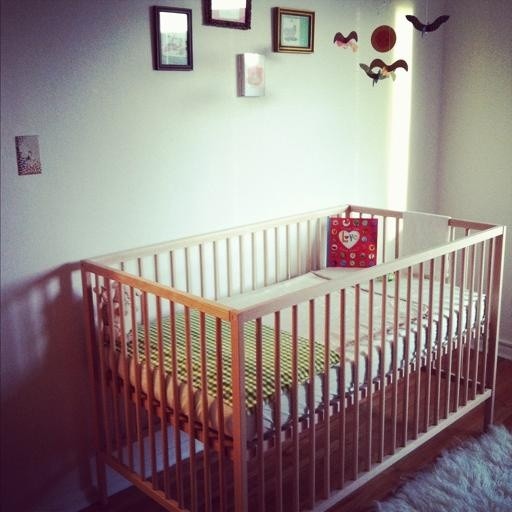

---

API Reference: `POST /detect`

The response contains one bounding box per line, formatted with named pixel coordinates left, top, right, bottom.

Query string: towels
left=397, top=209, right=452, bottom=280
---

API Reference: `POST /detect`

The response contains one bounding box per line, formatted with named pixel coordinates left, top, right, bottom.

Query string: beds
left=76, top=201, right=508, bottom=512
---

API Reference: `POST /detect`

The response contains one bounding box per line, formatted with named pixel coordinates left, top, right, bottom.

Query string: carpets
left=371, top=418, right=512, bottom=512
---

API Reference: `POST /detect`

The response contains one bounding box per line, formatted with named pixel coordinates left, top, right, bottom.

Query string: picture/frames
left=149, top=3, right=196, bottom=73
left=202, top=0, right=254, bottom=30
left=270, top=4, right=317, bottom=53
